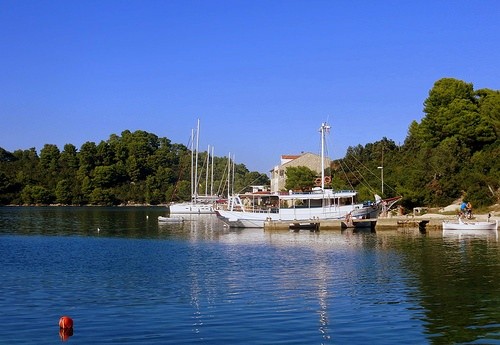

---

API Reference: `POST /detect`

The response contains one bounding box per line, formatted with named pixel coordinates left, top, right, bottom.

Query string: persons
left=466, top=201, right=472, bottom=218
left=461, top=202, right=469, bottom=216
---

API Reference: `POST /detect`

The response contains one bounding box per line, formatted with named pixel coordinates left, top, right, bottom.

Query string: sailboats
left=156, top=116, right=403, bottom=229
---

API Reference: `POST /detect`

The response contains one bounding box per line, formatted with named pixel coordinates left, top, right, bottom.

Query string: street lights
left=377, top=166, right=384, bottom=196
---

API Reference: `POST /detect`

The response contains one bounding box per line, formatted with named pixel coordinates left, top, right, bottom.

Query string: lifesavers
left=324, top=176, right=331, bottom=184
left=314, top=178, right=322, bottom=185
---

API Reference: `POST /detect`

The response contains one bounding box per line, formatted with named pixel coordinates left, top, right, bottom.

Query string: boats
left=442, top=213, right=499, bottom=230
